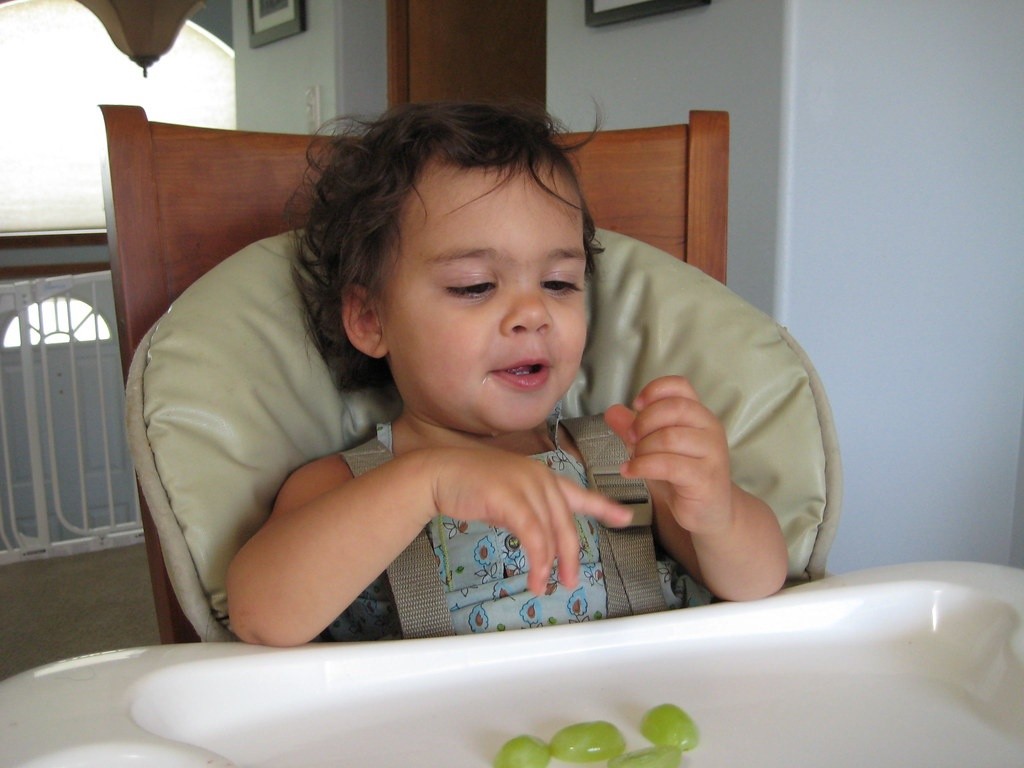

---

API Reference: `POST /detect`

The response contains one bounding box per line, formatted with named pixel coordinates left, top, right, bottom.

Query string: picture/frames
left=584, top=0, right=712, bottom=27
left=247, top=0, right=307, bottom=50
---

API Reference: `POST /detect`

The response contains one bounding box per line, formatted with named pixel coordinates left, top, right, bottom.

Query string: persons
left=227, top=100, right=788, bottom=649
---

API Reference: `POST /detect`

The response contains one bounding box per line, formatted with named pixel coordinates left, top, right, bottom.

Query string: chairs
left=98, top=102, right=732, bottom=634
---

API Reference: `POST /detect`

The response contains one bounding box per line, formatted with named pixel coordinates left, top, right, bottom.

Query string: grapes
left=490, top=702, right=699, bottom=768
